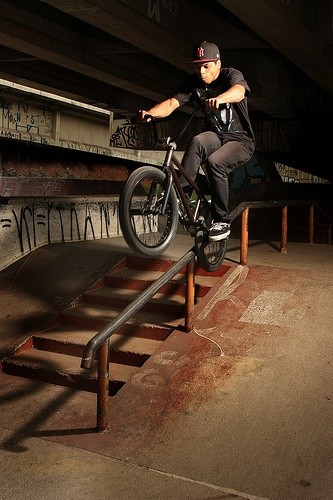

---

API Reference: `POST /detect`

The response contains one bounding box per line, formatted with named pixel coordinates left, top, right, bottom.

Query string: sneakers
left=208, top=219, right=230, bottom=241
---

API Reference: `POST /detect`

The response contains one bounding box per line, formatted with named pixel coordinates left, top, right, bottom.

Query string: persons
left=138, top=41, right=256, bottom=243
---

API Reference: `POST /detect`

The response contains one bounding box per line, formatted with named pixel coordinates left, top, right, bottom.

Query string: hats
left=193, top=40, right=220, bottom=63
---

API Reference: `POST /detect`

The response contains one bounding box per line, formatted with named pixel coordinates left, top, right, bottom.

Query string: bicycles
left=120, top=100, right=230, bottom=271
